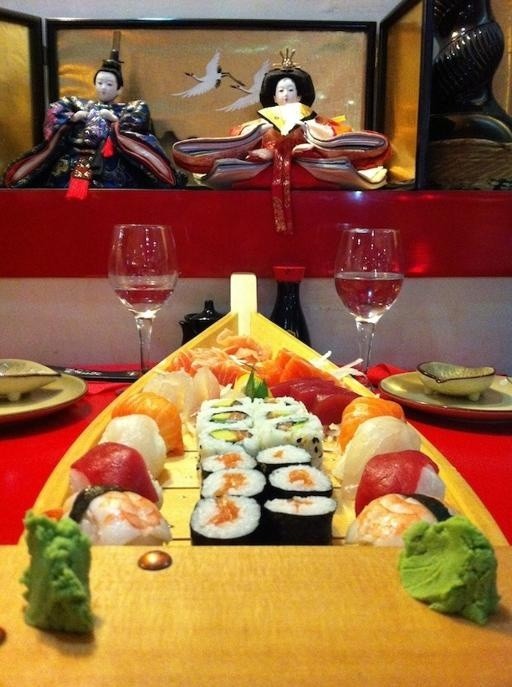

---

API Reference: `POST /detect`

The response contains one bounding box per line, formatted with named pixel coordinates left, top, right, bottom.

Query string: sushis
left=190, top=394, right=339, bottom=544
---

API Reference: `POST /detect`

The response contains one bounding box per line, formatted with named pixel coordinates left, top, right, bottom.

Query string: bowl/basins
left=417, top=361, right=496, bottom=401
left=1, top=358, right=60, bottom=401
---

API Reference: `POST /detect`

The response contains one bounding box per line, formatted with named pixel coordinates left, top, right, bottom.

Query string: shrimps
left=346, top=493, right=460, bottom=545
left=59, top=491, right=173, bottom=546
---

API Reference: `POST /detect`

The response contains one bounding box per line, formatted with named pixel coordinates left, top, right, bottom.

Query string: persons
left=1, top=49, right=190, bottom=200
left=168, top=49, right=394, bottom=189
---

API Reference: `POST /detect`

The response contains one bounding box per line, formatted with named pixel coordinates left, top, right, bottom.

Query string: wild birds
left=215, top=56, right=275, bottom=114
left=169, top=50, right=248, bottom=98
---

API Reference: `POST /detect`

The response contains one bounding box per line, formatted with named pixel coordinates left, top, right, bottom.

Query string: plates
left=380, top=372, right=511, bottom=423
left=0, top=373, right=88, bottom=427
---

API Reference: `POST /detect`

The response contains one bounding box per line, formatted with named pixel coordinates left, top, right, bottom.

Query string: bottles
left=270, top=264, right=311, bottom=345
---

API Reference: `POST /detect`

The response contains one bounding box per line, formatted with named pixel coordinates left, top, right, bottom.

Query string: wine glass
left=108, top=224, right=178, bottom=370
left=333, top=226, right=405, bottom=369
